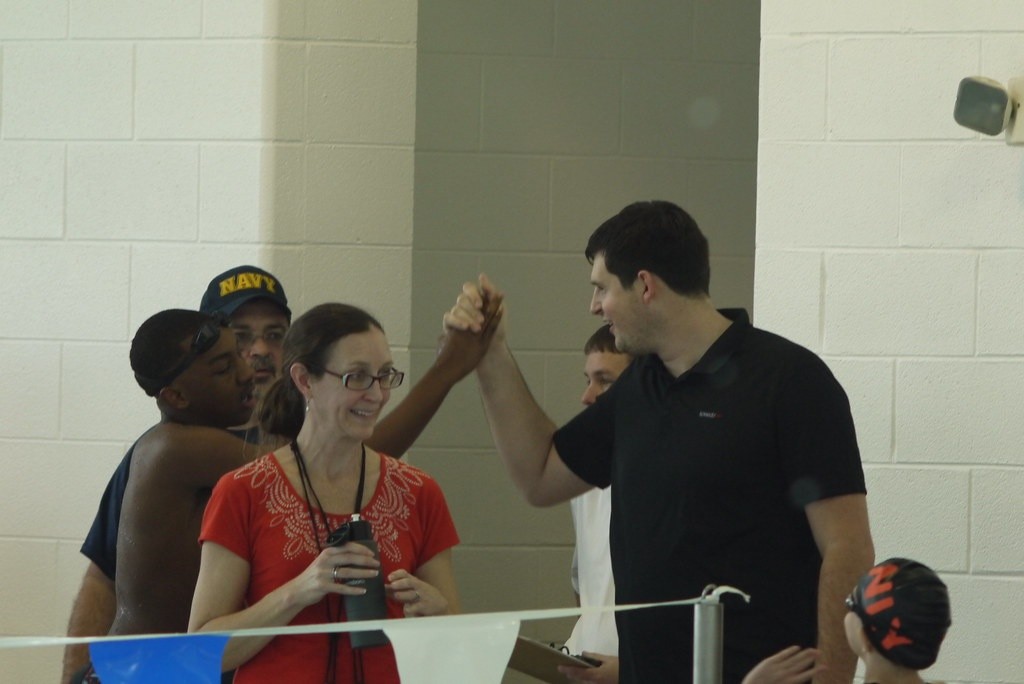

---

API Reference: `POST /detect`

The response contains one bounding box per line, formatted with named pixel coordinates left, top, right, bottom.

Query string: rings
left=332, top=566, right=339, bottom=578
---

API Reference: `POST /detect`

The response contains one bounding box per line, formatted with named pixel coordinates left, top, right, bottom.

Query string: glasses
left=162, top=312, right=230, bottom=375
left=845, top=590, right=858, bottom=616
left=308, top=364, right=407, bottom=392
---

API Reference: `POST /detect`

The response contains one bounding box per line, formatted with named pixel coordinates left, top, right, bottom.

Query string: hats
left=200, top=265, right=291, bottom=321
left=858, top=558, right=953, bottom=669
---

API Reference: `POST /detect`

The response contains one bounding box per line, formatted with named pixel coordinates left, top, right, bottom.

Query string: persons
left=442, top=200, right=876, bottom=684
left=60, top=265, right=504, bottom=684
left=557, top=323, right=619, bottom=684
left=741, top=558, right=951, bottom=684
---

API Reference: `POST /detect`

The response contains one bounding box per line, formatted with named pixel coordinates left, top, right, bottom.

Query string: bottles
left=327, top=512, right=390, bottom=648
